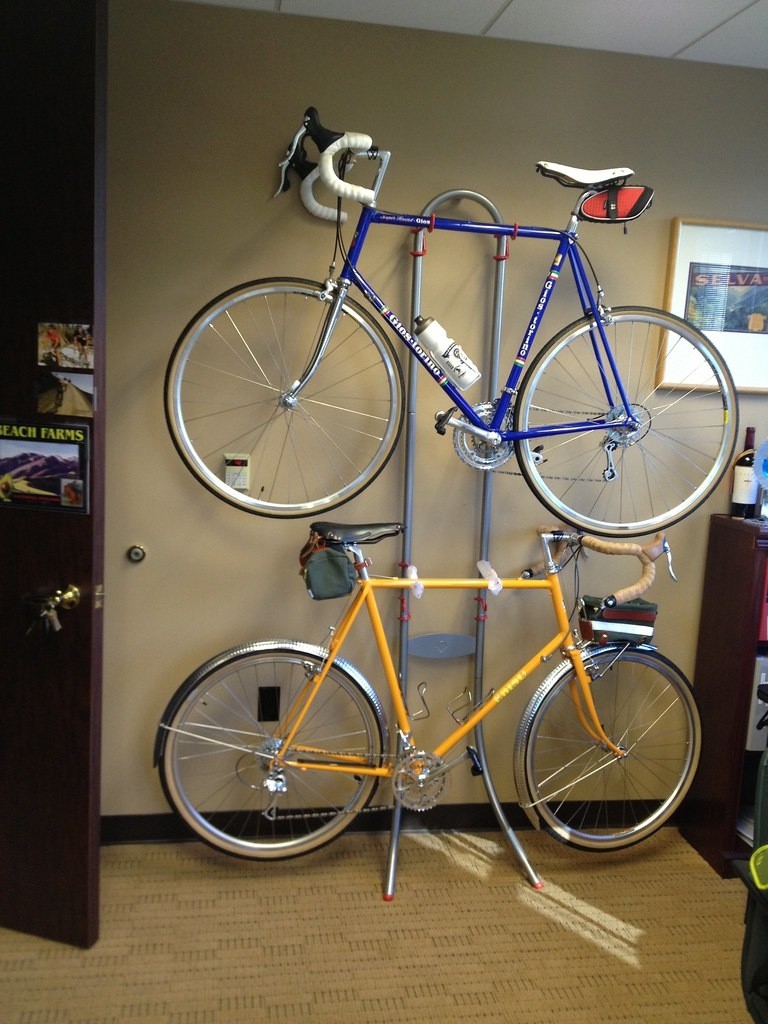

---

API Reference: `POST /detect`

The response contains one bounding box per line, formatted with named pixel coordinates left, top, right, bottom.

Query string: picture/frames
left=655, top=215, right=768, bottom=393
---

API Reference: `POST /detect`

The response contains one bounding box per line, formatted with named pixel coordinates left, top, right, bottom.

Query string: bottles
left=414, top=316, right=480, bottom=390
left=731, top=427, right=759, bottom=518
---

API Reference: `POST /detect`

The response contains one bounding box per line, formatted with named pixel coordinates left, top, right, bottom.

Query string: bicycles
left=146, top=517, right=703, bottom=864
left=161, top=103, right=742, bottom=540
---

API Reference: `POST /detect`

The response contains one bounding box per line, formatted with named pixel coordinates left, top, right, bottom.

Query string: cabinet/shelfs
left=688, top=514, right=768, bottom=877
